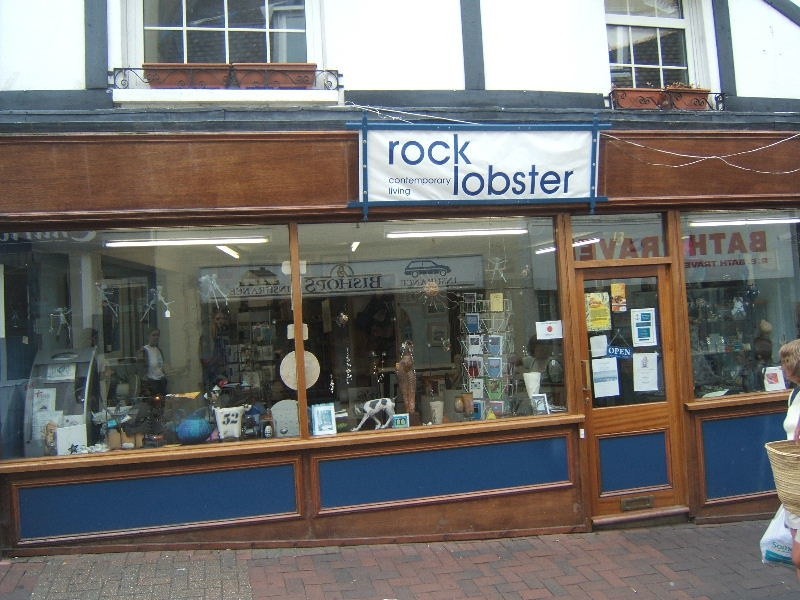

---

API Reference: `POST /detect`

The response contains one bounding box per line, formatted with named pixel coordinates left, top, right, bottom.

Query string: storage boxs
left=108, top=428, right=144, bottom=449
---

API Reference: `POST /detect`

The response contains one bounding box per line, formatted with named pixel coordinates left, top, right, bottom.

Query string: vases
left=232, top=62, right=317, bottom=88
left=141, top=63, right=231, bottom=89
left=612, top=88, right=665, bottom=109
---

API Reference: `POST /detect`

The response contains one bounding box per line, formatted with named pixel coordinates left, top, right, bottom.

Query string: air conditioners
left=384, top=227, right=529, bottom=239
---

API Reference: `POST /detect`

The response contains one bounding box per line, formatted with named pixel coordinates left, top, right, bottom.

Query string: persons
left=518, top=336, right=563, bottom=415
left=199, top=308, right=236, bottom=402
left=80, top=331, right=113, bottom=443
left=780, top=338, right=800, bottom=577
left=136, top=328, right=166, bottom=432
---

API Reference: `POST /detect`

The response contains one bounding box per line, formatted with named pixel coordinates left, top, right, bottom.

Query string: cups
left=430, top=400, right=443, bottom=425
left=455, top=396, right=463, bottom=413
left=523, top=372, right=540, bottom=400
left=462, top=392, right=474, bottom=416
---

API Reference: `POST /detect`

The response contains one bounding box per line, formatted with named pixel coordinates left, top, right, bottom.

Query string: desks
left=378, top=362, right=457, bottom=425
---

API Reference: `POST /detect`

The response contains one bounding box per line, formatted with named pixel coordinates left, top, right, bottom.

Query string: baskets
left=765, top=441, right=800, bottom=517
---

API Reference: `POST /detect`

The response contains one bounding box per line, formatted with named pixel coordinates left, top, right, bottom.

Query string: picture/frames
left=313, top=406, right=337, bottom=435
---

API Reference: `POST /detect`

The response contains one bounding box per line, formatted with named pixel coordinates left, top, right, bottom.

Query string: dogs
left=350, top=393, right=403, bottom=432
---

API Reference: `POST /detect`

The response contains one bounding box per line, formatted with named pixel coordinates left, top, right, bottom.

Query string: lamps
left=216, top=244, right=240, bottom=259
left=687, top=213, right=800, bottom=227
left=102, top=235, right=269, bottom=244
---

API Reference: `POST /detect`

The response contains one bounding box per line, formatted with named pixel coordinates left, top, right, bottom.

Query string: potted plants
left=665, top=81, right=711, bottom=110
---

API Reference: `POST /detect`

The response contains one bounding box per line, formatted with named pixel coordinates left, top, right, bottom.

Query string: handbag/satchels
left=760, top=502, right=794, bottom=570
